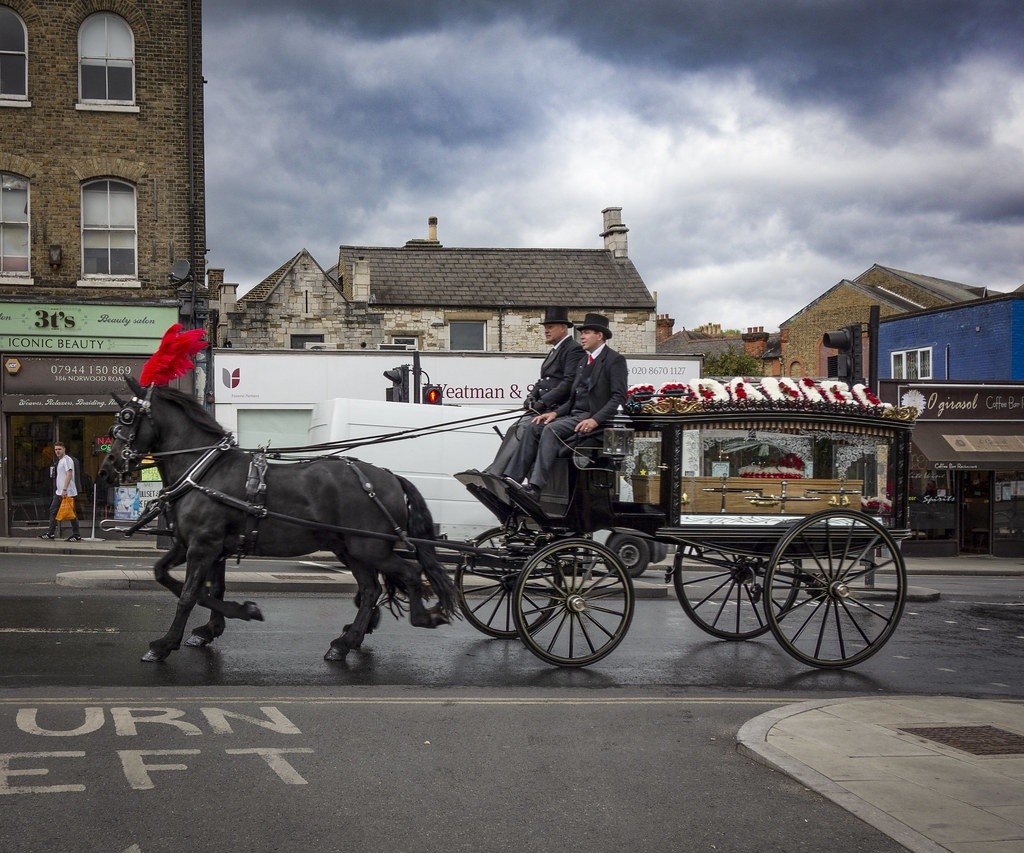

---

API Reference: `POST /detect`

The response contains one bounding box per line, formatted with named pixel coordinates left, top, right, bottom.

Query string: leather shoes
left=504, top=477, right=541, bottom=503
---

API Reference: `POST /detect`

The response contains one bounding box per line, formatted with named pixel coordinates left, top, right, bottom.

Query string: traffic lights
left=823, top=325, right=863, bottom=384
left=422, top=386, right=442, bottom=404
left=384, top=368, right=409, bottom=402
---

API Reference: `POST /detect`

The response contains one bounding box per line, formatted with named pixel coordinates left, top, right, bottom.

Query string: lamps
left=601, top=403, right=636, bottom=466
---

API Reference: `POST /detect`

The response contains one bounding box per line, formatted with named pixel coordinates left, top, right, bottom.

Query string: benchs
left=522, top=422, right=624, bottom=496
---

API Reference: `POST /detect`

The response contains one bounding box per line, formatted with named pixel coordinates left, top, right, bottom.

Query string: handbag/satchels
left=55, top=497, right=76, bottom=520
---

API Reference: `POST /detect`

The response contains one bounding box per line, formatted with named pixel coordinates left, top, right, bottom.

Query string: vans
left=308, top=398, right=668, bottom=577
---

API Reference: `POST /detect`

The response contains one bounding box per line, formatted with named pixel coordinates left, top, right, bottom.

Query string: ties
left=542, top=348, right=555, bottom=367
left=589, top=355, right=593, bottom=364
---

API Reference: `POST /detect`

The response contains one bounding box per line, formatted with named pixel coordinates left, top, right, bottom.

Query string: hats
left=539, top=306, right=574, bottom=328
left=576, top=313, right=612, bottom=339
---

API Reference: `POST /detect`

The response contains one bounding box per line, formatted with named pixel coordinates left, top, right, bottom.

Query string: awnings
left=912, top=424, right=1024, bottom=470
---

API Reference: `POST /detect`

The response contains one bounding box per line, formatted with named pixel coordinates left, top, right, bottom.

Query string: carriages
left=93, top=373, right=926, bottom=670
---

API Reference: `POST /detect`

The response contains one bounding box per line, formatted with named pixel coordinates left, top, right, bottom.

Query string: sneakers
left=64, top=535, right=82, bottom=542
left=37, top=533, right=55, bottom=541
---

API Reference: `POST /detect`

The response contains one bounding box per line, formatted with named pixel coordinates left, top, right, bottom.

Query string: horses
left=96, top=373, right=467, bottom=665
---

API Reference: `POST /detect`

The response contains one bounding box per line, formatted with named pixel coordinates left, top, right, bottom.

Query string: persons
left=489, top=306, right=628, bottom=502
left=38, top=441, right=82, bottom=542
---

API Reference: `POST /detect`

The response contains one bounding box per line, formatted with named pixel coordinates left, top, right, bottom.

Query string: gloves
left=533, top=399, right=547, bottom=413
left=524, top=397, right=536, bottom=410
